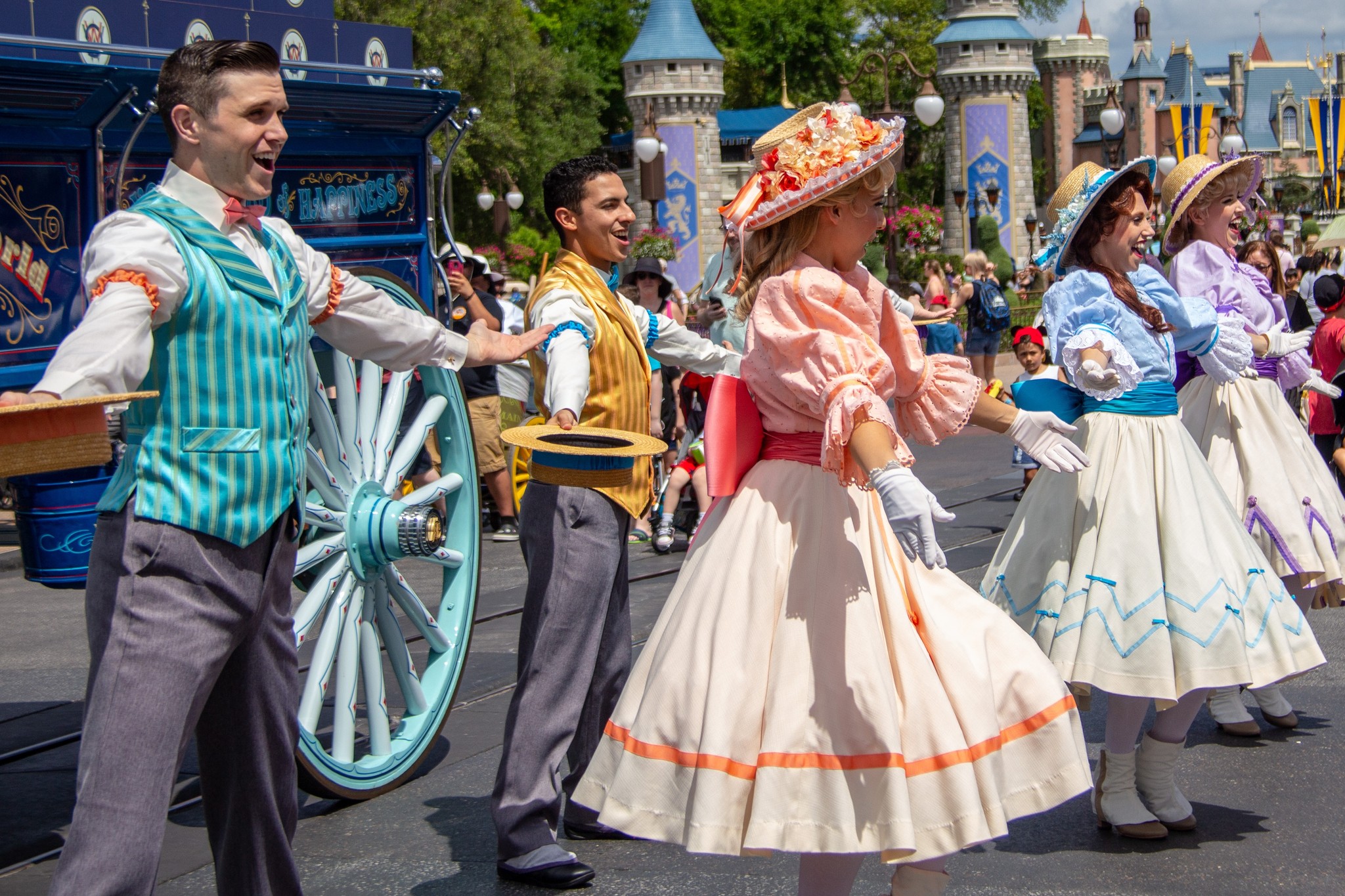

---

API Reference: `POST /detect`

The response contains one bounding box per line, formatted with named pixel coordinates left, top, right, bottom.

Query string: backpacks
left=966, top=274, right=1011, bottom=332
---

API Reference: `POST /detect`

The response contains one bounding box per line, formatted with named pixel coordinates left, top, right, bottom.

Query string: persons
left=857, top=248, right=1018, bottom=427
left=491, top=156, right=745, bottom=890
left=989, top=326, right=1069, bottom=502
left=0, top=42, right=558, bottom=896
left=978, top=155, right=1326, bottom=839
left=355, top=242, right=532, bottom=543
left=1235, top=228, right=1345, bottom=502
left=1146, top=233, right=1160, bottom=257
left=1006, top=264, right=1065, bottom=306
left=1161, top=154, right=1345, bottom=734
left=614, top=200, right=749, bottom=556
left=572, top=101, right=1095, bottom=895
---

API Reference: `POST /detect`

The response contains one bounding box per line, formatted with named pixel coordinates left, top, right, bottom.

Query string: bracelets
left=464, top=291, right=475, bottom=302
left=1004, top=396, right=1013, bottom=405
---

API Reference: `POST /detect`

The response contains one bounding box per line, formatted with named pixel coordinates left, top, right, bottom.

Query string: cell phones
left=448, top=260, right=464, bottom=278
left=709, top=296, right=723, bottom=310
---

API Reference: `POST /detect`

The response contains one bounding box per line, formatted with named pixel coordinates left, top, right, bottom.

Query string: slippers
left=627, top=527, right=652, bottom=545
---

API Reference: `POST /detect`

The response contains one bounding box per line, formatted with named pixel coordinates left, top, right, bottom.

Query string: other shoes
left=491, top=516, right=520, bottom=542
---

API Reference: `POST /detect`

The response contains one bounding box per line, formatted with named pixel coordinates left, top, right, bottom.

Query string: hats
left=1313, top=274, right=1345, bottom=312
left=1012, top=327, right=1046, bottom=362
left=622, top=257, right=672, bottom=299
left=704, top=100, right=906, bottom=295
left=474, top=254, right=504, bottom=283
left=0, top=390, right=160, bottom=479
left=1032, top=154, right=1158, bottom=282
left=437, top=242, right=486, bottom=280
left=930, top=295, right=949, bottom=309
left=1160, top=153, right=1268, bottom=256
left=498, top=425, right=669, bottom=488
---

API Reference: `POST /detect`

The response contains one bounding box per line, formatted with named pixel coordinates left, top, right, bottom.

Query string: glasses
left=637, top=272, right=658, bottom=280
left=1255, top=264, right=1272, bottom=277
left=719, top=225, right=729, bottom=236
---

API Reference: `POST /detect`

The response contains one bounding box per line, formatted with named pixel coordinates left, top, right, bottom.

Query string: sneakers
left=687, top=520, right=699, bottom=544
left=655, top=520, right=675, bottom=547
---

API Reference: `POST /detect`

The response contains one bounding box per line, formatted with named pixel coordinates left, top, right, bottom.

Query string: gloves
left=1259, top=318, right=1312, bottom=361
left=866, top=460, right=956, bottom=570
left=1300, top=368, right=1342, bottom=398
left=1238, top=365, right=1259, bottom=378
left=1079, top=359, right=1121, bottom=392
left=1001, top=407, right=1093, bottom=474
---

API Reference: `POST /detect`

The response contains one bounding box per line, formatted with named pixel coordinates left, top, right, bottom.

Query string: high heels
left=1215, top=719, right=1260, bottom=736
left=1240, top=685, right=1298, bottom=728
left=1158, top=813, right=1197, bottom=830
left=1090, top=813, right=1169, bottom=839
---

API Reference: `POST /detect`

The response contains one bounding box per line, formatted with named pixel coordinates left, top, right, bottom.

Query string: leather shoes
left=497, top=859, right=595, bottom=890
left=562, top=820, right=633, bottom=840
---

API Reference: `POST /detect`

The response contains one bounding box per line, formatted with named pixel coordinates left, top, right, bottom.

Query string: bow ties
left=222, top=197, right=267, bottom=232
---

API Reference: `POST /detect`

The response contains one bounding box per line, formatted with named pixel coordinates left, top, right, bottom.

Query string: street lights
left=477, top=166, right=526, bottom=237
left=1216, top=111, right=1250, bottom=164
left=1096, top=82, right=1130, bottom=173
left=635, top=104, right=670, bottom=233
left=837, top=51, right=946, bottom=294
